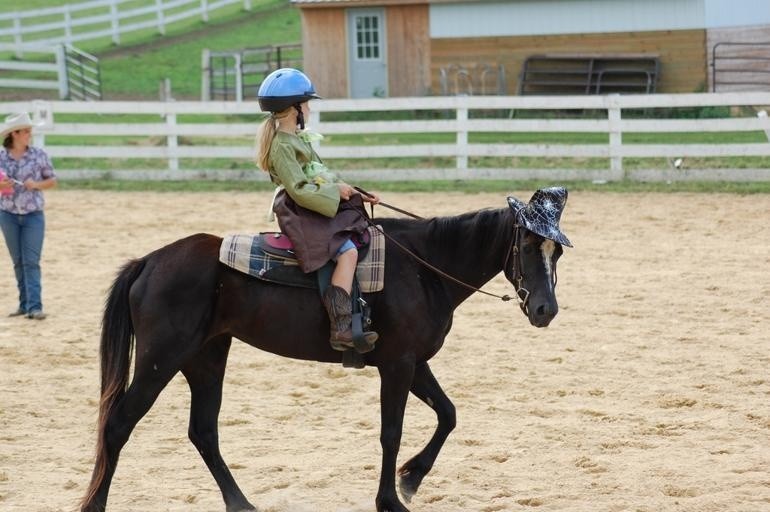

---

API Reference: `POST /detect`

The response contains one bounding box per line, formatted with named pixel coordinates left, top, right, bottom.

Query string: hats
left=1, top=112, right=45, bottom=136
left=507, top=186, right=574, bottom=248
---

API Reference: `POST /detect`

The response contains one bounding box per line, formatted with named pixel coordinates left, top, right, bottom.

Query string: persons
left=254, top=66, right=380, bottom=355
left=1, top=111, right=58, bottom=322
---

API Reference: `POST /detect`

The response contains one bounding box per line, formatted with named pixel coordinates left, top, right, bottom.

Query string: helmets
left=257, top=68, right=323, bottom=113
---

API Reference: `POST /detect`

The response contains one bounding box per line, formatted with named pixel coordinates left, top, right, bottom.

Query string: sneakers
left=9, top=309, right=26, bottom=317
left=28, top=311, right=50, bottom=320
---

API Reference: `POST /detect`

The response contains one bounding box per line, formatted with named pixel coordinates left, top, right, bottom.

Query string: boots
left=323, top=284, right=380, bottom=352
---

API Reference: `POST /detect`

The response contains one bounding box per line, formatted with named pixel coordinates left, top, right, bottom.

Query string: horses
left=76, top=205, right=564, bottom=512
